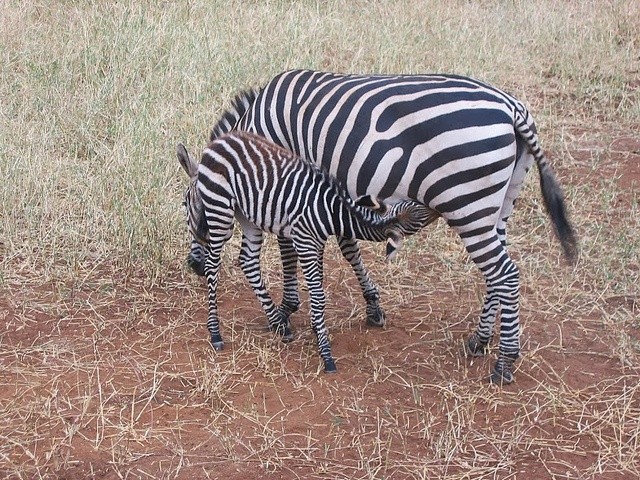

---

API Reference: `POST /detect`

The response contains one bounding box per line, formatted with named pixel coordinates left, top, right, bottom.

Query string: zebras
left=174, top=68, right=582, bottom=386
